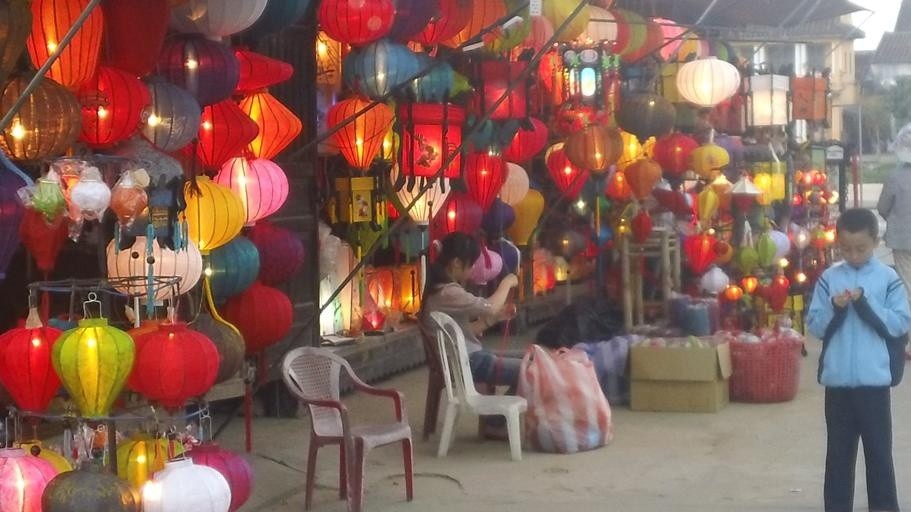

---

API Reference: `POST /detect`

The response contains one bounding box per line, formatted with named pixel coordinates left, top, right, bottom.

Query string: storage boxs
left=629, top=335, right=736, bottom=410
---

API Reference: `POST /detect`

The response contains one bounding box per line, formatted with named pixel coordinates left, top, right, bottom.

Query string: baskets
left=731, top=340, right=800, bottom=403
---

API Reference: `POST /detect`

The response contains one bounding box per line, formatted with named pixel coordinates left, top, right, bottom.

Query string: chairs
left=278, top=345, right=417, bottom=511
left=407, top=311, right=499, bottom=438
left=428, top=307, right=526, bottom=464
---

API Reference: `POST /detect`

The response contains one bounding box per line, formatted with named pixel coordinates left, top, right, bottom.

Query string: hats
left=887, top=125, right=910, bottom=164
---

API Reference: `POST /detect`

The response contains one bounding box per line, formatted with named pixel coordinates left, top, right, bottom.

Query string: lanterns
left=1, top=428, right=251, bottom=512
left=316, top=1, right=844, bottom=336
left=1, top=2, right=304, bottom=417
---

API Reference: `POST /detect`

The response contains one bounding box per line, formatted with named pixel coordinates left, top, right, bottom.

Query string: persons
left=807, top=207, right=911, bottom=512
left=422, top=232, right=533, bottom=439
left=877, top=123, right=911, bottom=360
